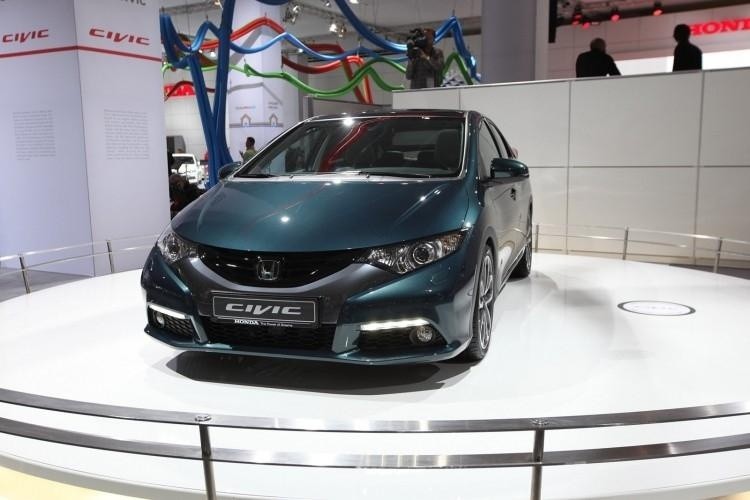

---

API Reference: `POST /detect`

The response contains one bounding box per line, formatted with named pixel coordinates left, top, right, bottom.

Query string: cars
left=171, top=153, right=202, bottom=186
left=141, top=109, right=533, bottom=367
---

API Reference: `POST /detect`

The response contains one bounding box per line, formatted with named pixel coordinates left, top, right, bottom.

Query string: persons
left=405, top=26, right=445, bottom=89
left=239, top=137, right=257, bottom=165
left=672, top=24, right=703, bottom=72
left=575, top=38, right=622, bottom=78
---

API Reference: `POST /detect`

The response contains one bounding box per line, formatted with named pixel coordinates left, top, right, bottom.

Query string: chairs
left=340, top=131, right=463, bottom=168
